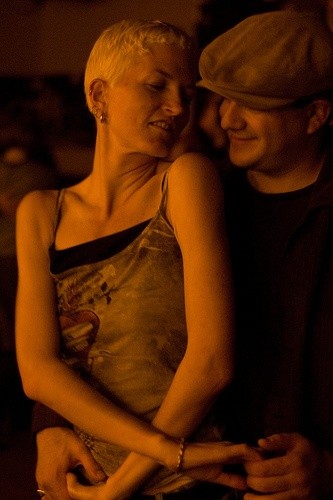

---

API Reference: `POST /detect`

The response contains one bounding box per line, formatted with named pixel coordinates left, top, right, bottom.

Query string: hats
left=195, top=9, right=332, bottom=110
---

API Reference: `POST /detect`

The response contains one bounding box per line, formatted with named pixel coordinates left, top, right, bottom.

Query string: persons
left=15, top=17, right=273, bottom=498
left=165, top=4, right=333, bottom=499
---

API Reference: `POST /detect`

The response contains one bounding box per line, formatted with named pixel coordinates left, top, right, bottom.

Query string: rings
left=37, top=489, right=46, bottom=498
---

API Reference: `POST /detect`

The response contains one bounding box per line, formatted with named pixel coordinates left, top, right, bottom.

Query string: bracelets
left=174, top=436, right=186, bottom=474
